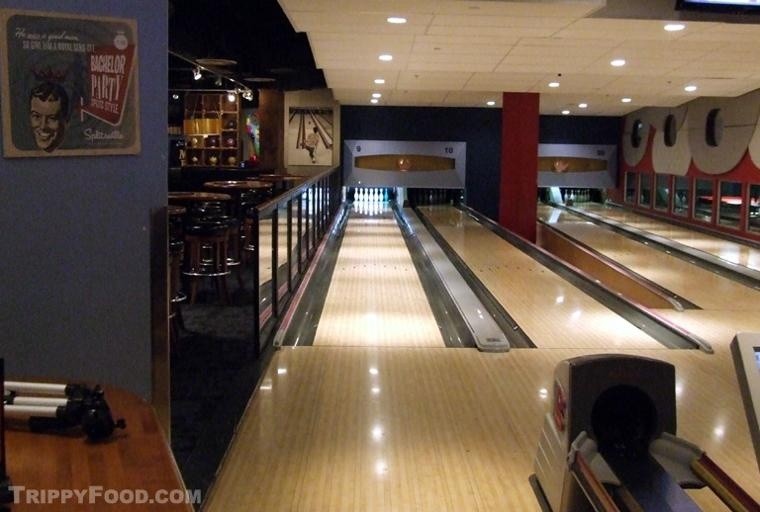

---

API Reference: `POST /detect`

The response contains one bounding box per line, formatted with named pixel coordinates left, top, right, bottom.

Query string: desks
left=168, top=174, right=283, bottom=237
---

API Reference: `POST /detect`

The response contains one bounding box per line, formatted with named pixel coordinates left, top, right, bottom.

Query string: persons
left=304, top=126, right=320, bottom=162
left=27, top=81, right=69, bottom=152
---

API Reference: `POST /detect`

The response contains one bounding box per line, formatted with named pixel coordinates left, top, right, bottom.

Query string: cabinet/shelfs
left=183, top=93, right=242, bottom=170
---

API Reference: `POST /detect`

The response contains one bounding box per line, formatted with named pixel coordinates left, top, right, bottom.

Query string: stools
left=170, top=189, right=273, bottom=357
